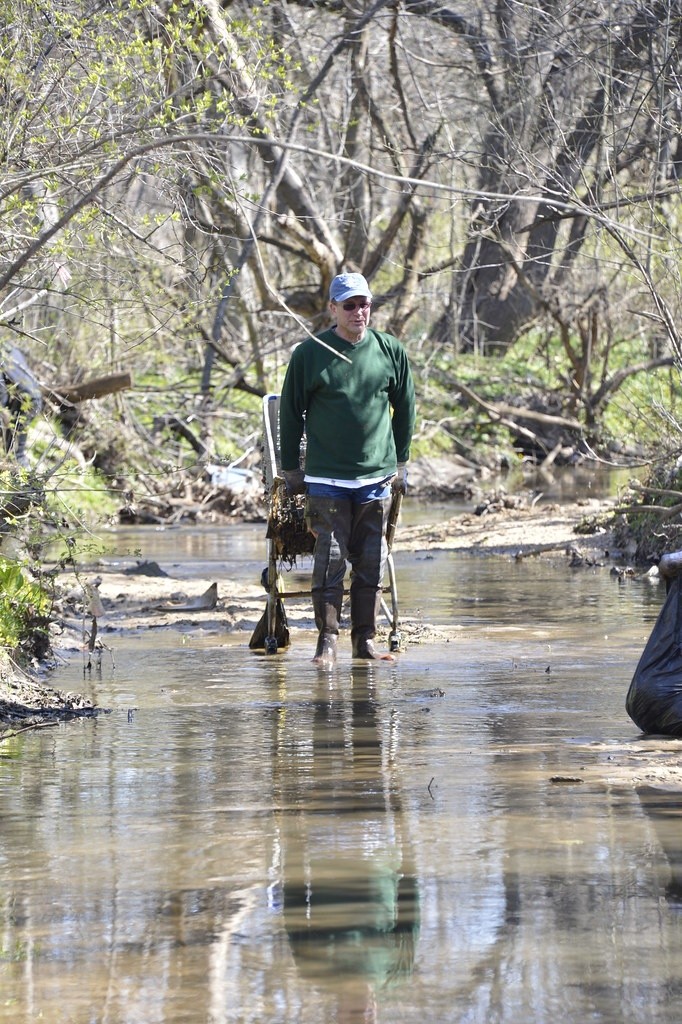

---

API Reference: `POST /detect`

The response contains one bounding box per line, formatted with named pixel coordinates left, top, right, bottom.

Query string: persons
left=279, top=274, right=416, bottom=664
left=282, top=665, right=421, bottom=1023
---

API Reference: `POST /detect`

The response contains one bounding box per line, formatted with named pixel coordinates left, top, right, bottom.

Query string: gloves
left=390, top=460, right=408, bottom=495
left=283, top=468, right=307, bottom=497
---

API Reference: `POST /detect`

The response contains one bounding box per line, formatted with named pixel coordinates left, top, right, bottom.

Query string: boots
left=347, top=493, right=396, bottom=662
left=301, top=495, right=352, bottom=664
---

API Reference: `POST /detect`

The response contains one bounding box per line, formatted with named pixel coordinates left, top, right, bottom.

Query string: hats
left=329, top=273, right=373, bottom=302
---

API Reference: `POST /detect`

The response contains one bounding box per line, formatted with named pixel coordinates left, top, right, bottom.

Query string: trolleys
left=247, top=390, right=409, bottom=656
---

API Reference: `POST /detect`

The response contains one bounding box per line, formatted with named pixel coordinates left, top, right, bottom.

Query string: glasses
left=335, top=300, right=372, bottom=311
